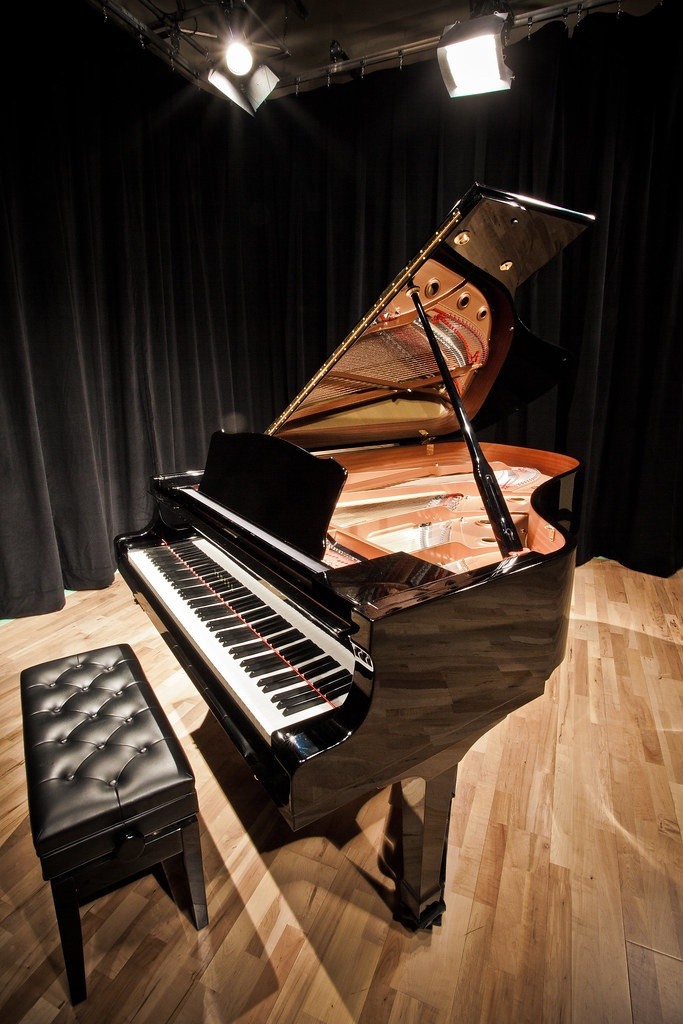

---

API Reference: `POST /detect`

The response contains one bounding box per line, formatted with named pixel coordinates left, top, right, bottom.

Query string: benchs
left=20, top=644, right=209, bottom=1008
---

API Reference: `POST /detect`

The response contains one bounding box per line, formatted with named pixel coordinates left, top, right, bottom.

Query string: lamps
left=437, top=12, right=517, bottom=98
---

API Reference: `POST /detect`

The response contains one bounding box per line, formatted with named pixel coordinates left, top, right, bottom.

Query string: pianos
left=113, top=175, right=600, bottom=934
